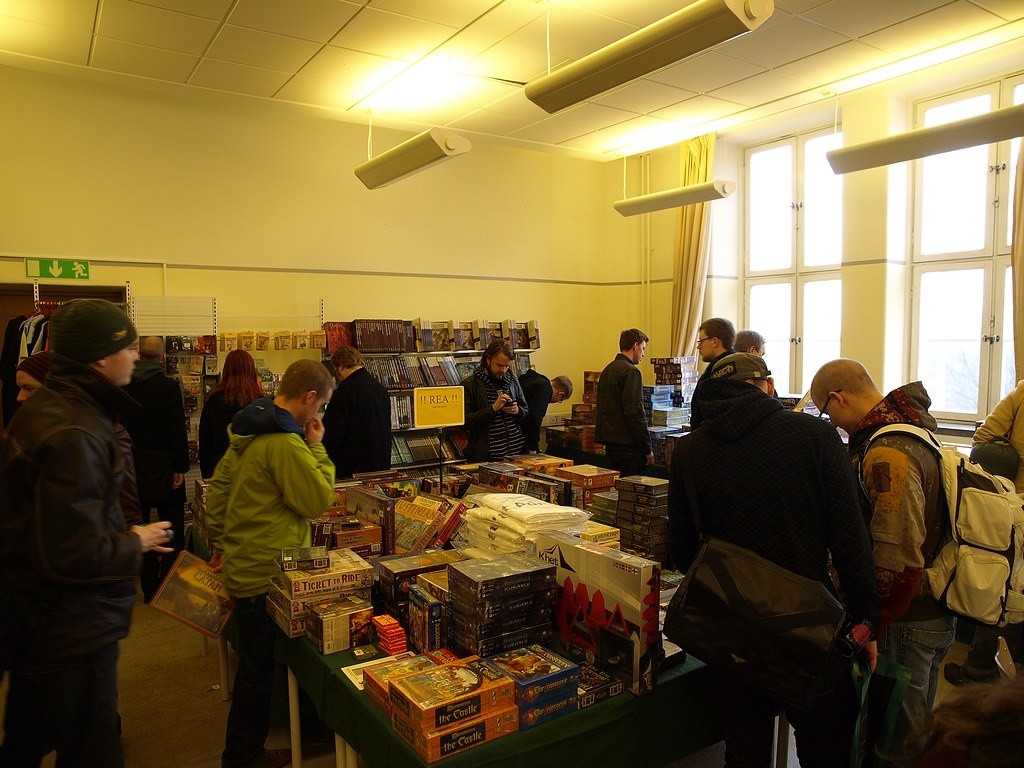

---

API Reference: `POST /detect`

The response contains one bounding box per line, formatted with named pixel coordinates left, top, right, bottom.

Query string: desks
left=186, top=528, right=783, bottom=767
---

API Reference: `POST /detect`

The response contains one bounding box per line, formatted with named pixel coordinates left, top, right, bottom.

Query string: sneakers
left=943, top=662, right=972, bottom=687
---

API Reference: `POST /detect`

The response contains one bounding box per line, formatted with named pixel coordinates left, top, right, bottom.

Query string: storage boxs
left=267, top=356, right=818, bottom=761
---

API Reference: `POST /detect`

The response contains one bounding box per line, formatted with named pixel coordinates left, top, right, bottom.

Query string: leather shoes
left=301, top=726, right=336, bottom=758
left=222, top=748, right=292, bottom=768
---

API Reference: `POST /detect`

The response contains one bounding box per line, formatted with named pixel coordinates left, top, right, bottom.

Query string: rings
left=167, top=530, right=174, bottom=539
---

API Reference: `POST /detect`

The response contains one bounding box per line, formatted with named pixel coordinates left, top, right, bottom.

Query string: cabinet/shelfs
left=322, top=347, right=541, bottom=471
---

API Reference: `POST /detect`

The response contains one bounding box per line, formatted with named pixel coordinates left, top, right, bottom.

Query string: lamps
left=825, top=93, right=1024, bottom=176
left=612, top=157, right=737, bottom=218
left=353, top=106, right=473, bottom=191
left=522, top=0, right=775, bottom=116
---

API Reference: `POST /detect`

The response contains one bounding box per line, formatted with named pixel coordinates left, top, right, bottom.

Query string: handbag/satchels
left=663, top=536, right=846, bottom=716
left=969, top=435, right=1019, bottom=483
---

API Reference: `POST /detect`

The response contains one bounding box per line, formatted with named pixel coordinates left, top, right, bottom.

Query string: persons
left=455, top=338, right=530, bottom=461
left=124, top=333, right=190, bottom=606
left=0, top=351, right=144, bottom=737
left=593, top=328, right=655, bottom=477
left=196, top=348, right=267, bottom=480
left=514, top=368, right=573, bottom=455
left=0, top=295, right=175, bottom=768
left=204, top=358, right=337, bottom=768
left=322, top=343, right=393, bottom=479
left=664, top=314, right=1024, bottom=768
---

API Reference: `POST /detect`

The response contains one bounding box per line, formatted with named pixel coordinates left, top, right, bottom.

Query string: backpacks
left=858, top=423, right=1024, bottom=627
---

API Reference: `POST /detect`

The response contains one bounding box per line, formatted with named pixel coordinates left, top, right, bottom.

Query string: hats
left=15, top=350, right=55, bottom=387
left=710, top=351, right=771, bottom=383
left=50, top=298, right=137, bottom=365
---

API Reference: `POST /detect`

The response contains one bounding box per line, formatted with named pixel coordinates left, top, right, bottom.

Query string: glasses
left=558, top=394, right=564, bottom=403
left=696, top=336, right=719, bottom=344
left=818, top=389, right=842, bottom=423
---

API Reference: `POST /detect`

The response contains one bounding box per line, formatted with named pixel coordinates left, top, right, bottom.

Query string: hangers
left=23, top=300, right=64, bottom=320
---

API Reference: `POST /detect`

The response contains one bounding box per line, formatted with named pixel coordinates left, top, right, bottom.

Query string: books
left=325, top=316, right=541, bottom=481
